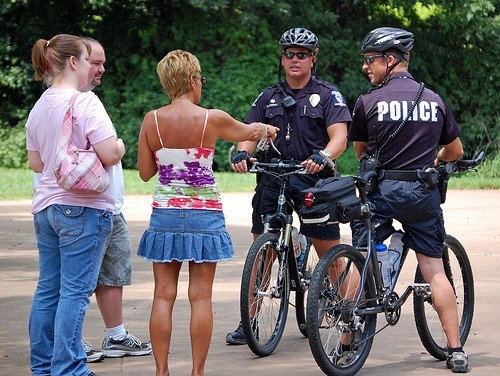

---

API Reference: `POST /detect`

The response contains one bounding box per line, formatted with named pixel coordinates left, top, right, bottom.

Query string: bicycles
left=228, top=145, right=330, bottom=357
left=306, top=150, right=486, bottom=376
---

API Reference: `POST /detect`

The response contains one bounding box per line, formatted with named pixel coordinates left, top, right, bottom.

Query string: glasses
left=193, top=76, right=206, bottom=84
left=365, top=54, right=383, bottom=65
left=284, top=52, right=310, bottom=60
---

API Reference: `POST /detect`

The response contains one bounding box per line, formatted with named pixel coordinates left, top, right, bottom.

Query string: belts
left=383, top=170, right=418, bottom=182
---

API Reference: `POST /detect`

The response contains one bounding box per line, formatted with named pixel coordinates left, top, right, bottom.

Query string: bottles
left=291, top=226, right=301, bottom=258
left=297, top=235, right=308, bottom=272
left=387, top=229, right=405, bottom=272
left=374, top=242, right=392, bottom=288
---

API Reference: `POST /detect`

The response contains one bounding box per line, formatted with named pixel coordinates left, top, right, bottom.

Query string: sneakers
left=81, top=338, right=105, bottom=363
left=226, top=318, right=259, bottom=344
left=102, top=332, right=152, bottom=357
left=446, top=351, right=469, bottom=373
left=329, top=342, right=359, bottom=366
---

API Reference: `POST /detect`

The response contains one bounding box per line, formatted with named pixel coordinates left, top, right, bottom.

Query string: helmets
left=359, top=27, right=414, bottom=56
left=280, top=28, right=319, bottom=56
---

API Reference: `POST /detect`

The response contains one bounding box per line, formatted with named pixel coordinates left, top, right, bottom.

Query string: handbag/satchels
left=53, top=93, right=111, bottom=195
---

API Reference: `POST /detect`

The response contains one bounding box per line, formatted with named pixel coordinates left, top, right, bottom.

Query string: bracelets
left=262, top=123, right=269, bottom=138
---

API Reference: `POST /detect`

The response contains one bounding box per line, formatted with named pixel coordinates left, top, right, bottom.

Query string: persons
left=137, top=50, right=281, bottom=376
left=34, top=38, right=152, bottom=363
left=350, top=27, right=469, bottom=373
left=25, top=34, right=125, bottom=376
left=226, top=27, right=359, bottom=366
